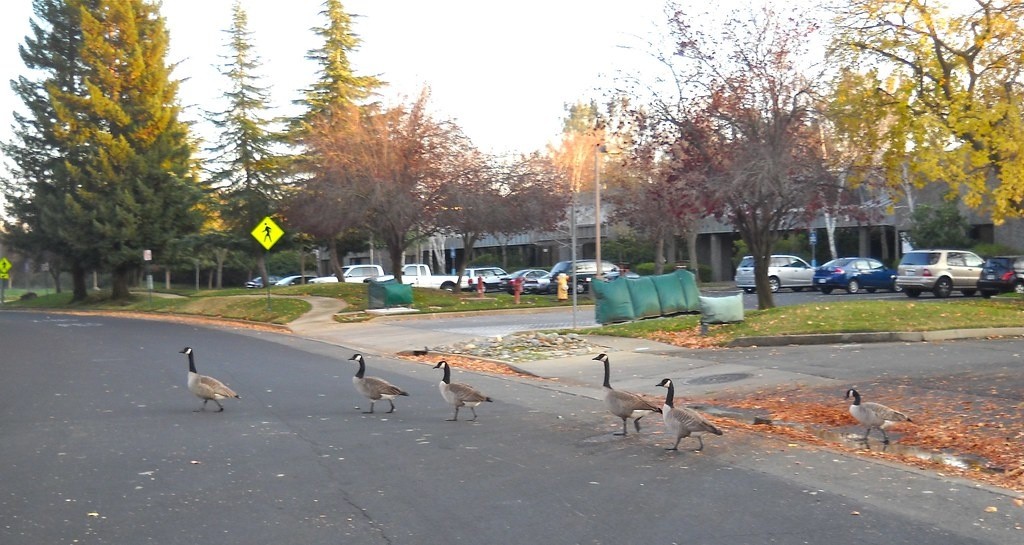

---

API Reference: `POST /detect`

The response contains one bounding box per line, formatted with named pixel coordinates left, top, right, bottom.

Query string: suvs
left=735, top=254, right=817, bottom=293
left=896, top=249, right=985, bottom=297
left=549, top=260, right=621, bottom=294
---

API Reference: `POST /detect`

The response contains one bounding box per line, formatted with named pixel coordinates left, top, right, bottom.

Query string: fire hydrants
left=554, top=273, right=571, bottom=304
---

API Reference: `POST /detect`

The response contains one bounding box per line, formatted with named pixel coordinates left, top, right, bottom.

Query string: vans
left=274, top=276, right=317, bottom=286
left=463, top=267, right=509, bottom=292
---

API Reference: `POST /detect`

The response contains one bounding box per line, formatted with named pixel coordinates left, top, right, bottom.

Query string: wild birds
left=844, top=390, right=912, bottom=444
left=592, top=354, right=663, bottom=436
left=432, top=361, right=493, bottom=422
left=179, top=347, right=243, bottom=413
left=348, top=353, right=409, bottom=413
left=656, top=378, right=723, bottom=452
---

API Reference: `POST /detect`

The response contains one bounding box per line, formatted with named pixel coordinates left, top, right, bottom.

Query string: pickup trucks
left=308, top=264, right=395, bottom=283
left=364, top=264, right=472, bottom=293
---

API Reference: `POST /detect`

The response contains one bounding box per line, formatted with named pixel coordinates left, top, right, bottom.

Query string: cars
left=813, top=257, right=903, bottom=293
left=976, top=255, right=1023, bottom=299
left=497, top=269, right=549, bottom=295
left=245, top=275, right=283, bottom=288
